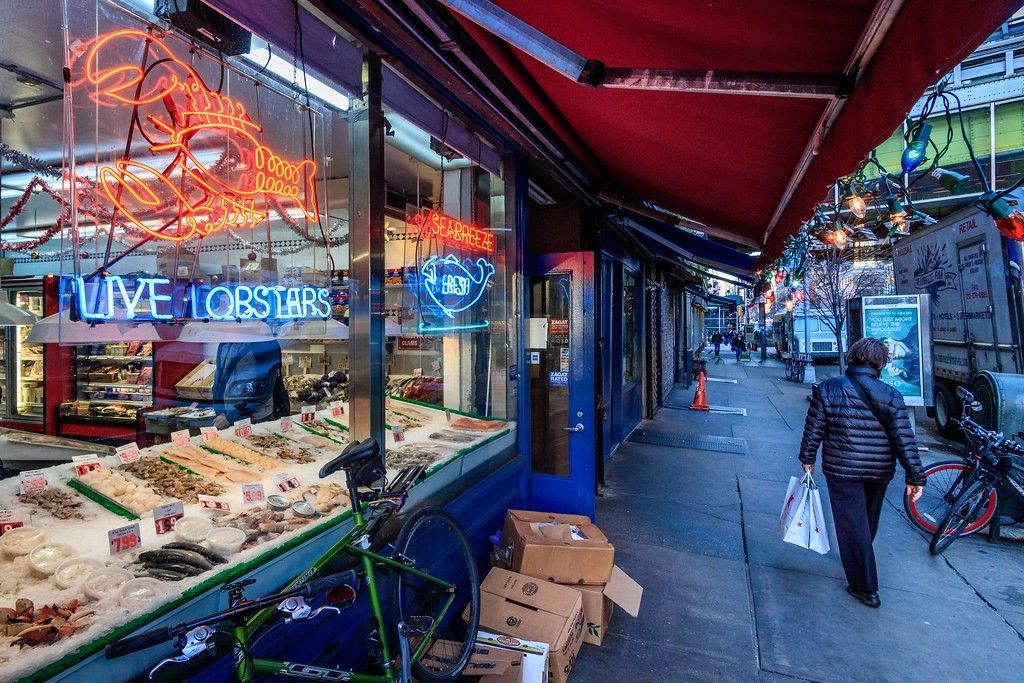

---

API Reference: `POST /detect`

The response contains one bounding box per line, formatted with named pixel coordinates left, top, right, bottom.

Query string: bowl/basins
left=170, top=516, right=213, bottom=543
left=0, top=526, right=48, bottom=562
left=206, top=527, right=246, bottom=555
left=52, top=557, right=170, bottom=613
left=28, top=541, right=79, bottom=579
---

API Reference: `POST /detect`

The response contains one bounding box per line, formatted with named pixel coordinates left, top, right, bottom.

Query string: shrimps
left=16, top=487, right=85, bottom=523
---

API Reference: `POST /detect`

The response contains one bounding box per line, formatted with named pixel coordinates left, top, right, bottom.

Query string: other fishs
left=133, top=542, right=230, bottom=582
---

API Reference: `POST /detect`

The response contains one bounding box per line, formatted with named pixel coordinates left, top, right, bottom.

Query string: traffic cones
left=689, top=371, right=711, bottom=411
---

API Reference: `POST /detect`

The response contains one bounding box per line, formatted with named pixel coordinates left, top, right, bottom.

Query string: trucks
left=893, top=193, right=1023, bottom=437
left=772, top=309, right=848, bottom=365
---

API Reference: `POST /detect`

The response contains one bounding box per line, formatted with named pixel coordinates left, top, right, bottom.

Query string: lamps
left=0, top=291, right=41, bottom=327
left=174, top=69, right=276, bottom=343
left=22, top=0, right=163, bottom=345
left=276, top=114, right=349, bottom=340
left=385, top=317, right=402, bottom=337
left=401, top=162, right=435, bottom=334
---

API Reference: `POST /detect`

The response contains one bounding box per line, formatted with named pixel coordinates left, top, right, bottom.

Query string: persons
left=211, top=289, right=288, bottom=431
left=880, top=323, right=922, bottom=387
left=797, top=337, right=927, bottom=607
left=709, top=330, right=744, bottom=361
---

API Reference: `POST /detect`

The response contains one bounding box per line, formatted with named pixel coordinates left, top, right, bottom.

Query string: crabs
left=0, top=597, right=97, bottom=653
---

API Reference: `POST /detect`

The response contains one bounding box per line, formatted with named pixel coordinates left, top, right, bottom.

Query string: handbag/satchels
left=731, top=347, right=735, bottom=352
left=778, top=469, right=830, bottom=555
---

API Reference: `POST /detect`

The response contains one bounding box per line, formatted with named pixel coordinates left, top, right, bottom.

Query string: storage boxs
left=394, top=636, right=523, bottom=683
left=155, top=245, right=200, bottom=279
left=475, top=630, right=550, bottom=683
left=206, top=265, right=239, bottom=288
left=175, top=357, right=216, bottom=400
left=566, top=565, right=643, bottom=647
left=301, top=271, right=325, bottom=286
left=500, top=508, right=615, bottom=586
left=88, top=367, right=120, bottom=383
left=240, top=258, right=278, bottom=285
left=0, top=258, right=14, bottom=275
left=461, top=567, right=588, bottom=683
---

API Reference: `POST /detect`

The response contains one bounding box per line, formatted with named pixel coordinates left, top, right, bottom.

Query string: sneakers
left=846, top=584, right=882, bottom=607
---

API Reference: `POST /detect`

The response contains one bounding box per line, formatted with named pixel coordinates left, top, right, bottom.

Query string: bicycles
left=785, top=352, right=805, bottom=383
left=106, top=438, right=481, bottom=683
left=899, top=387, right=1024, bottom=554
